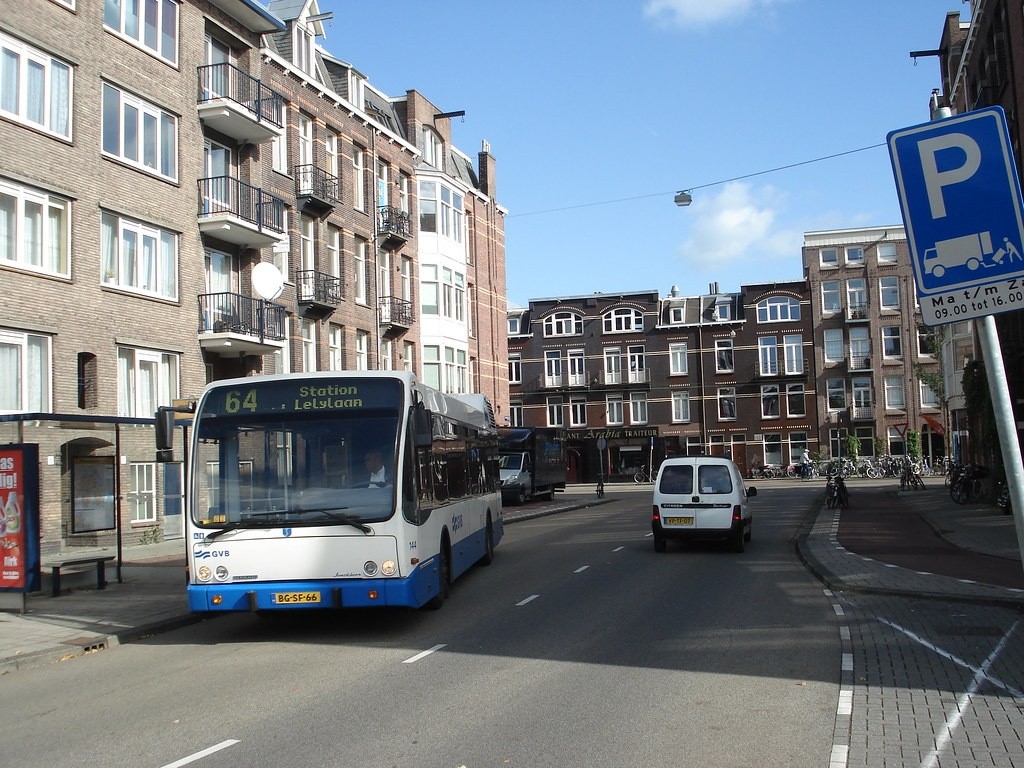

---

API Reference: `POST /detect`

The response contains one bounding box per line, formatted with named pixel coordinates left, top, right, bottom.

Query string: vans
left=652, top=456, right=757, bottom=553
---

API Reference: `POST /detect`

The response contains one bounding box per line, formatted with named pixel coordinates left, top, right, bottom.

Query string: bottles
left=5, top=492, right=20, bottom=534
left=0, top=496, right=6, bottom=538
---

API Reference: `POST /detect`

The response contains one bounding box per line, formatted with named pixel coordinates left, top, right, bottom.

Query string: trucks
left=497, top=426, right=567, bottom=505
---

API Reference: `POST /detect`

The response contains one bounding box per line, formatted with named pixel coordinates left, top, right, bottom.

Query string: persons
left=800, top=449, right=811, bottom=478
left=359, top=449, right=393, bottom=487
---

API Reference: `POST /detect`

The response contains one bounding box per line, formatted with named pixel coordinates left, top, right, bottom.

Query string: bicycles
left=596, top=472, right=607, bottom=498
left=634, top=464, right=658, bottom=484
left=746, top=455, right=1011, bottom=517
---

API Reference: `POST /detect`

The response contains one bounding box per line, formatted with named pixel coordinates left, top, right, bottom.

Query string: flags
left=920, top=415, right=944, bottom=435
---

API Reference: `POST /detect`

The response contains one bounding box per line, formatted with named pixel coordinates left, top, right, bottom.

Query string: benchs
left=45, top=555, right=116, bottom=596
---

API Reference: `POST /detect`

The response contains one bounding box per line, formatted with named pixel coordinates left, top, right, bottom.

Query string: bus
left=154, top=371, right=504, bottom=619
